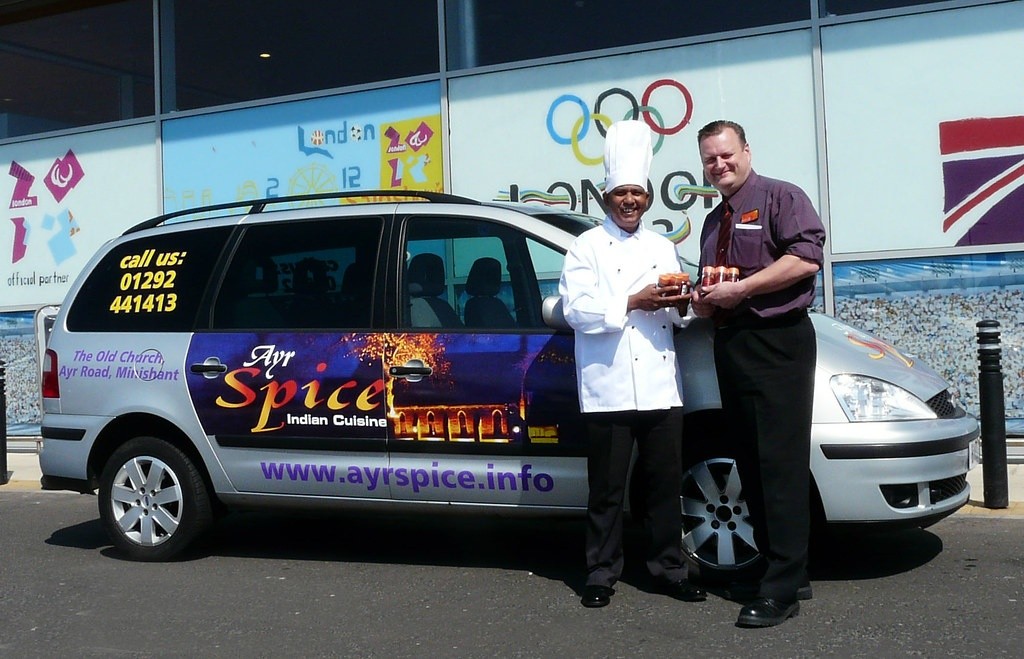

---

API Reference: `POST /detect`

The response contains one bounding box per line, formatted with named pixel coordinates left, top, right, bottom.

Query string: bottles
left=700, top=266, right=739, bottom=296
left=654, top=273, right=691, bottom=308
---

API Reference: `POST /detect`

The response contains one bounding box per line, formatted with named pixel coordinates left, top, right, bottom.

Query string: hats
left=603, top=120, right=653, bottom=195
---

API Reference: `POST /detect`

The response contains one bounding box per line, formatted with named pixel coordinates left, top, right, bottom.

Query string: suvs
left=40, top=190, right=981, bottom=586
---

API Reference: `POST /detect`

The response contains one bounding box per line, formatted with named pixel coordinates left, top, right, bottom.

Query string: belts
left=714, top=309, right=808, bottom=331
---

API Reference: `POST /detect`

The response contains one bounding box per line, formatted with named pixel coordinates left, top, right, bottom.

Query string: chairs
left=406, top=253, right=465, bottom=329
left=461, top=257, right=517, bottom=327
left=288, top=259, right=371, bottom=330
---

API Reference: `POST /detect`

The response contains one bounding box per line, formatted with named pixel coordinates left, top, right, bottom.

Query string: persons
left=692, top=120, right=825, bottom=629
left=557, top=119, right=706, bottom=607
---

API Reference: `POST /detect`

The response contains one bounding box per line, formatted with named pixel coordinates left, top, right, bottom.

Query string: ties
left=710, top=202, right=729, bottom=328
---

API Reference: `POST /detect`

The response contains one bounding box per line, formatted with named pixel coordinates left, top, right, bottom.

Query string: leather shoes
left=666, top=578, right=706, bottom=601
left=726, top=581, right=812, bottom=603
left=739, top=591, right=800, bottom=626
left=580, top=584, right=611, bottom=606
